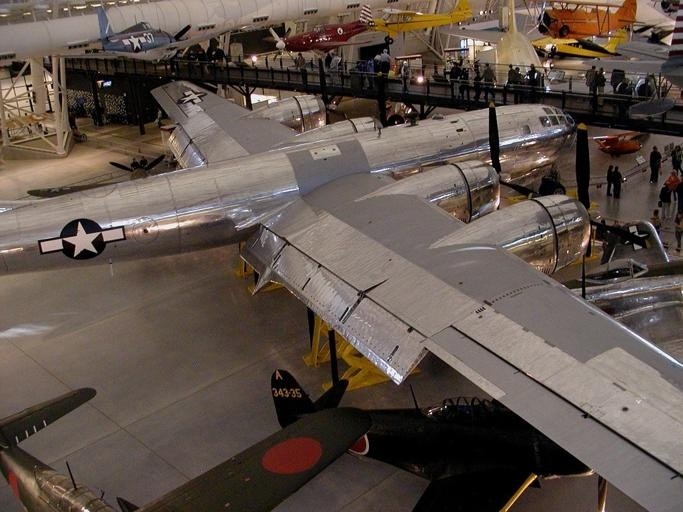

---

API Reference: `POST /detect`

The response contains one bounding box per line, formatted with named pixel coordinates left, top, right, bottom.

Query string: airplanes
left=530, top=0, right=638, bottom=61
left=92, top=2, right=252, bottom=65
left=267, top=0, right=476, bottom=64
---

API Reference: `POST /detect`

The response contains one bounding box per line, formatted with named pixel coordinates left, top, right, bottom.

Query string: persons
left=659, top=145, right=683, bottom=222
left=611, top=166, right=625, bottom=200
left=386, top=97, right=410, bottom=125
left=650, top=208, right=662, bottom=237
left=324, top=52, right=341, bottom=86
left=671, top=213, right=682, bottom=253
left=388, top=60, right=412, bottom=94
left=605, top=165, right=613, bottom=197
left=612, top=220, right=621, bottom=228
left=449, top=55, right=497, bottom=102
left=649, top=145, right=662, bottom=185
left=349, top=49, right=392, bottom=89
left=585, top=66, right=606, bottom=95
left=196, top=48, right=210, bottom=74
left=613, top=77, right=652, bottom=120
left=505, top=64, right=541, bottom=104
left=296, top=53, right=307, bottom=86
left=228, top=57, right=236, bottom=67
left=551, top=44, right=556, bottom=57
